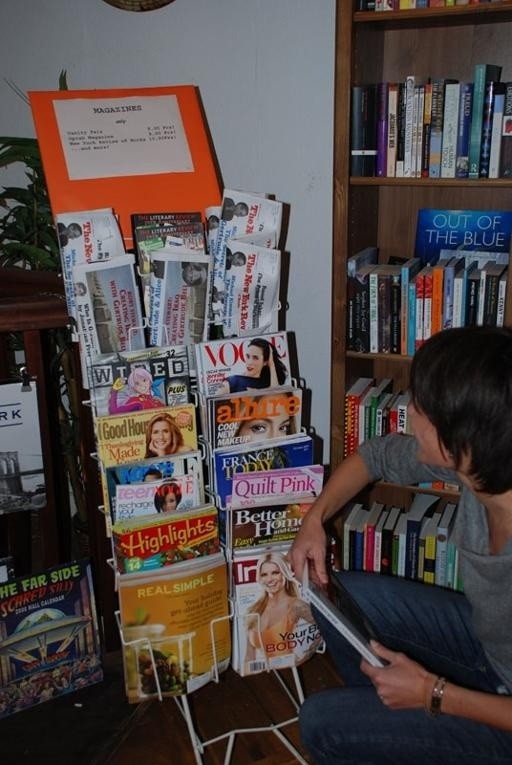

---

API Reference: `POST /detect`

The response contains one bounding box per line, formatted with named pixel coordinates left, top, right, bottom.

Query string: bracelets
left=430, top=676, right=448, bottom=721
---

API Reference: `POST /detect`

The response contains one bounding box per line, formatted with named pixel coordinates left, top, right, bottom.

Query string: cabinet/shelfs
left=0, top=295, right=122, bottom=653
left=329, top=0, right=512, bottom=572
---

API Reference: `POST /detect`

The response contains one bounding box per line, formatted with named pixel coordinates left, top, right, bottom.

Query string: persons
left=282, top=323, right=512, bottom=765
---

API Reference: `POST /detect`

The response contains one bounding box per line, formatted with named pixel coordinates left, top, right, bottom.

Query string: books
left=302, top=582, right=383, bottom=667
left=334, top=0, right=511, bottom=593
left=2, top=187, right=325, bottom=720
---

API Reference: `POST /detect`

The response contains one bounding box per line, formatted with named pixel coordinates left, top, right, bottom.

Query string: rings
left=380, top=696, right=383, bottom=702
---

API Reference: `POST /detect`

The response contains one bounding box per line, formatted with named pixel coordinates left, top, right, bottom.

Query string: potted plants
left=0, top=135, right=62, bottom=295
